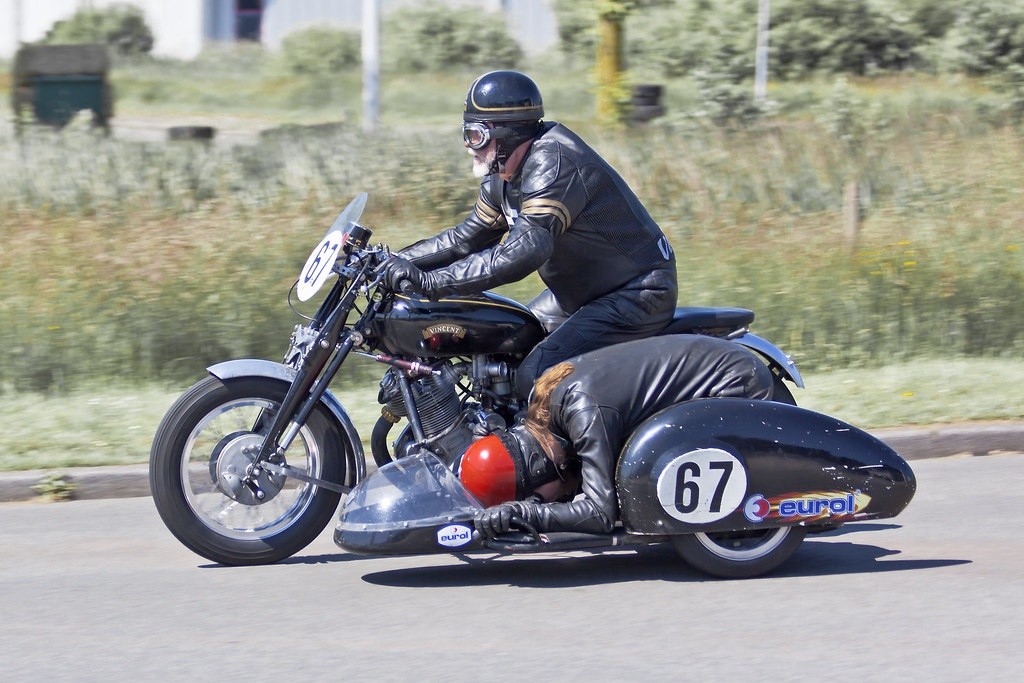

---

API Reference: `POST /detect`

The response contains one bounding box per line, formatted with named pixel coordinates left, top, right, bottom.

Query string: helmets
left=462, top=70, right=544, bottom=123
left=458, top=434, right=517, bottom=507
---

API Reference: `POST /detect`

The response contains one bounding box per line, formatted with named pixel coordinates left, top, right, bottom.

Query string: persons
left=376, top=71, right=678, bottom=407
left=456, top=334, right=773, bottom=535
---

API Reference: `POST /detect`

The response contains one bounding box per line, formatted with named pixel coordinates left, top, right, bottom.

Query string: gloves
left=373, top=256, right=425, bottom=301
left=475, top=500, right=522, bottom=539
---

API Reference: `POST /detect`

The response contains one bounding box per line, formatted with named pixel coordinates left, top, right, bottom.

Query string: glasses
left=461, top=121, right=493, bottom=151
left=524, top=493, right=545, bottom=505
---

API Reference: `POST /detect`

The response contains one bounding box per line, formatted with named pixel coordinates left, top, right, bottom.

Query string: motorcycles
left=148, top=190, right=918, bottom=580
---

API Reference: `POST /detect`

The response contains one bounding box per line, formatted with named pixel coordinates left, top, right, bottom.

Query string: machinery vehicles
left=11, top=40, right=113, bottom=130
